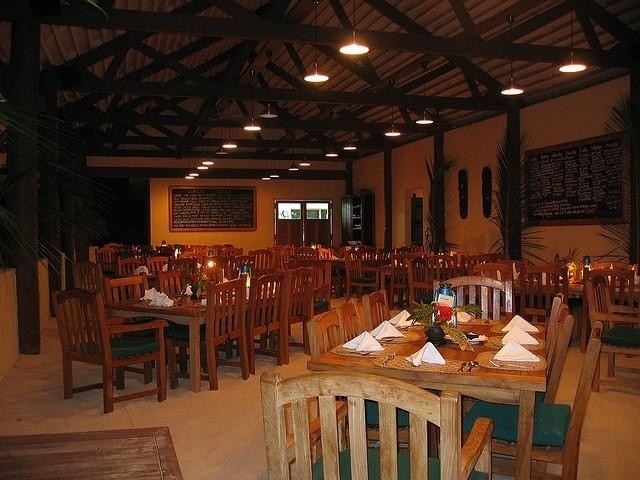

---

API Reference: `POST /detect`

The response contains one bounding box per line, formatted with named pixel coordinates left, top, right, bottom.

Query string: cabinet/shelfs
left=341, top=196, right=374, bottom=247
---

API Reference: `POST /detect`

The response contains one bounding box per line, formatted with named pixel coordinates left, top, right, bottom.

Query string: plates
left=444, top=333, right=487, bottom=345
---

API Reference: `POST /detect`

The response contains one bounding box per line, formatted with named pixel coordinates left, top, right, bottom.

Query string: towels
left=150, top=292, right=173, bottom=307
left=369, top=320, right=404, bottom=339
left=144, top=288, right=160, bottom=299
left=342, top=331, right=384, bottom=351
left=181, top=284, right=193, bottom=296
left=406, top=342, right=446, bottom=366
left=502, top=315, right=525, bottom=331
left=502, top=327, right=525, bottom=345
left=389, top=309, right=420, bottom=328
left=494, top=340, right=525, bottom=362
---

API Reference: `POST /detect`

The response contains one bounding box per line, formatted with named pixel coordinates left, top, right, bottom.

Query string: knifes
left=458, top=363, right=466, bottom=373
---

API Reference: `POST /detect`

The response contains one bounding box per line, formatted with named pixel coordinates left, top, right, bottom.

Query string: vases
left=422, top=325, right=451, bottom=346
left=188, top=282, right=203, bottom=302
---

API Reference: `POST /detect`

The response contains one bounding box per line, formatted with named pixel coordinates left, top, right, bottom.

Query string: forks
left=382, top=354, right=394, bottom=368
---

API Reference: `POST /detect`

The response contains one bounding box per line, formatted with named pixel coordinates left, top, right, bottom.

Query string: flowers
left=173, top=262, right=222, bottom=308
left=406, top=295, right=483, bottom=352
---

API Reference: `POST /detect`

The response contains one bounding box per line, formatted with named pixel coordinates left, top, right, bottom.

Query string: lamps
left=415, top=53, right=437, bottom=126
left=303, top=0, right=331, bottom=85
left=269, top=147, right=281, bottom=179
left=339, top=0, right=370, bottom=58
left=382, top=78, right=404, bottom=139
left=259, top=46, right=279, bottom=120
left=298, top=131, right=311, bottom=167
left=341, top=103, right=359, bottom=151
left=500, top=13, right=526, bottom=96
left=557, top=6, right=589, bottom=73
left=288, top=134, right=300, bottom=173
left=241, top=68, right=262, bottom=134
left=222, top=98, right=238, bottom=150
left=323, top=108, right=339, bottom=159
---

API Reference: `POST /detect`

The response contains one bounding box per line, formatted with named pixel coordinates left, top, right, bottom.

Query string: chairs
left=146, top=255, right=173, bottom=273
left=366, top=287, right=390, bottom=328
left=51, top=287, right=173, bottom=415
left=307, top=311, right=428, bottom=456
left=389, top=252, right=433, bottom=312
left=247, top=247, right=274, bottom=279
left=546, top=292, right=566, bottom=363
left=472, top=262, right=517, bottom=317
left=335, top=300, right=364, bottom=342
left=94, top=248, right=119, bottom=276
left=201, top=267, right=224, bottom=300
left=157, top=268, right=206, bottom=376
left=286, top=257, right=332, bottom=322
left=202, top=253, right=235, bottom=362
left=292, top=247, right=320, bottom=263
left=460, top=320, right=603, bottom=480
left=432, top=275, right=516, bottom=323
left=518, top=261, right=566, bottom=322
left=265, top=245, right=290, bottom=265
left=107, top=241, right=123, bottom=257
left=232, top=256, right=251, bottom=368
left=278, top=267, right=316, bottom=359
left=584, top=272, right=640, bottom=392
left=102, top=273, right=172, bottom=389
left=531, top=316, right=575, bottom=480
left=165, top=272, right=253, bottom=392
left=243, top=268, right=289, bottom=368
left=341, top=247, right=381, bottom=299
left=73, top=260, right=104, bottom=297
left=259, top=367, right=495, bottom=480
left=545, top=303, right=571, bottom=382
left=120, top=258, right=144, bottom=275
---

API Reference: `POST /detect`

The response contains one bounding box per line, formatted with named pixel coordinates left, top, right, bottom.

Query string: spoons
left=468, top=361, right=479, bottom=372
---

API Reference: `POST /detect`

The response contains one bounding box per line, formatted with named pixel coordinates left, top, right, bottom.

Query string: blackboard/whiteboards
left=524, top=128, right=630, bottom=226
left=169, top=186, right=257, bottom=231
